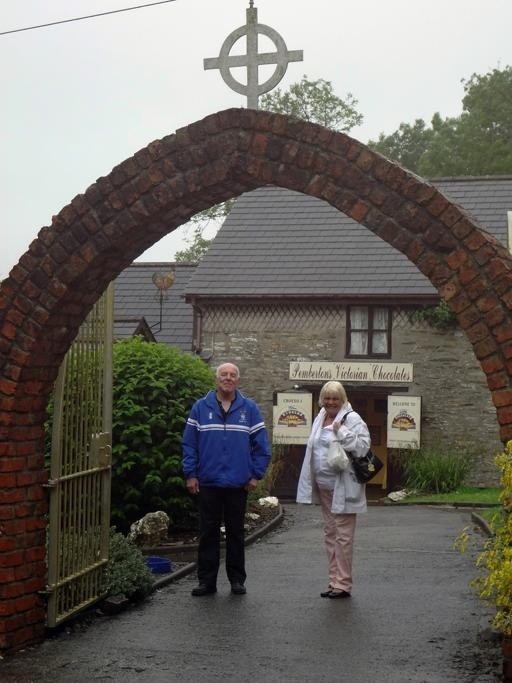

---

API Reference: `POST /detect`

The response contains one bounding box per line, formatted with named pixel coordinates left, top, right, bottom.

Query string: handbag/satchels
left=344, top=449, right=383, bottom=482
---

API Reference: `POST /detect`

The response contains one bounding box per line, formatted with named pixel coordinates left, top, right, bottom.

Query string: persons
left=180, top=362, right=273, bottom=597
left=294, top=380, right=372, bottom=600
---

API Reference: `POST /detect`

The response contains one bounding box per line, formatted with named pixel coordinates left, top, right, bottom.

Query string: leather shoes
left=321, top=589, right=350, bottom=598
left=231, top=582, right=246, bottom=594
left=192, top=584, right=217, bottom=596
